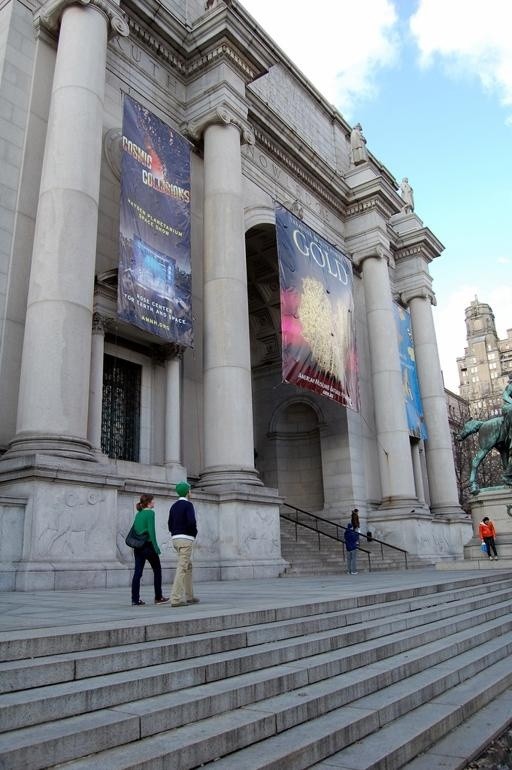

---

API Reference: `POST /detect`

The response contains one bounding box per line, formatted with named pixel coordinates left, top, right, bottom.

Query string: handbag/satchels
left=125, top=526, right=149, bottom=548
left=481, top=544, right=487, bottom=552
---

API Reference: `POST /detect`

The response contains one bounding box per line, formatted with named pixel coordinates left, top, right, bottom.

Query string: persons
left=479, top=516, right=498, bottom=561
left=351, top=508, right=361, bottom=551
left=344, top=523, right=359, bottom=576
left=400, top=176, right=415, bottom=212
left=130, top=494, right=170, bottom=606
left=165, top=480, right=201, bottom=608
left=350, top=122, right=370, bottom=166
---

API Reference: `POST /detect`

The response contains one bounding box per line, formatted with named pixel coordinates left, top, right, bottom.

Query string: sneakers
left=488, top=556, right=498, bottom=560
left=171, top=597, right=199, bottom=606
left=133, top=600, right=145, bottom=606
left=155, top=597, right=169, bottom=604
left=351, top=571, right=358, bottom=574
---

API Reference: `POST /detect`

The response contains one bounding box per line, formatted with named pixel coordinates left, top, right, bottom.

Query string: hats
left=176, top=481, right=191, bottom=495
left=354, top=509, right=359, bottom=512
left=348, top=523, right=353, bottom=528
